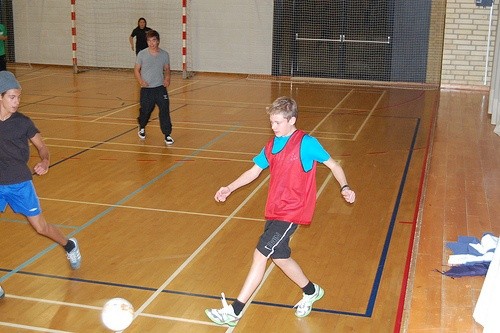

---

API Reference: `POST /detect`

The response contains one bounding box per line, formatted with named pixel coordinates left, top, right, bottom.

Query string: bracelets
left=340, top=185, right=349, bottom=193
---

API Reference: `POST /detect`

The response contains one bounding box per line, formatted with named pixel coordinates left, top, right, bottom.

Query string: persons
left=205, top=95, right=356, bottom=328
left=129, top=17, right=153, bottom=75
left=134, top=30, right=174, bottom=144
left=0, top=70, right=83, bottom=299
left=0, top=21, right=9, bottom=69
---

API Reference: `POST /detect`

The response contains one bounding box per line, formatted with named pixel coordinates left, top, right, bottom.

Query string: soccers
left=102, top=298, right=134, bottom=330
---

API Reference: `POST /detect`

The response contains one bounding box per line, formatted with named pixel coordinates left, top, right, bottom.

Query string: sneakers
left=205, top=292, right=240, bottom=327
left=137, top=127, right=145, bottom=139
left=0, top=286, right=5, bottom=298
left=164, top=136, right=174, bottom=144
left=66, top=238, right=81, bottom=268
left=293, top=283, right=324, bottom=317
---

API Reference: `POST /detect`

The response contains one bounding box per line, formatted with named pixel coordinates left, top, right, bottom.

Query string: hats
left=0, top=71, right=21, bottom=93
